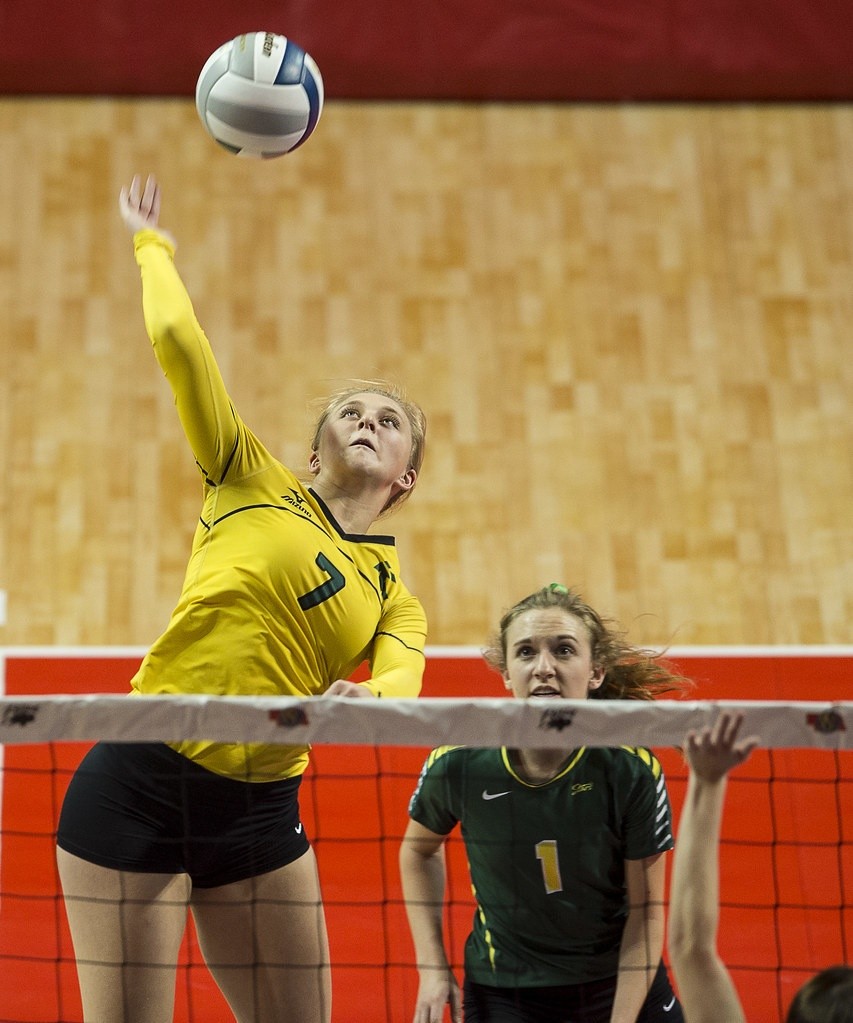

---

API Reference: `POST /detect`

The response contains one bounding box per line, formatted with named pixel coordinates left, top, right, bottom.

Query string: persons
left=398, top=584, right=853, bottom=1023
left=52, top=175, right=430, bottom=1022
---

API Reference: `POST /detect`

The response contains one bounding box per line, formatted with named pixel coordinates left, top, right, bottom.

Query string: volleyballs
left=195, top=30, right=324, bottom=160
